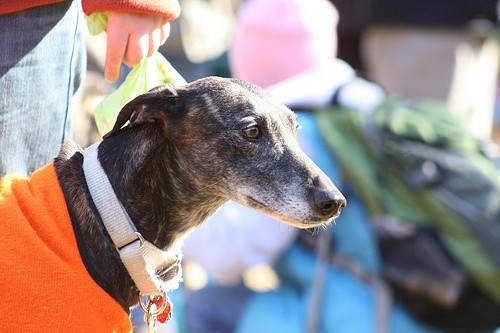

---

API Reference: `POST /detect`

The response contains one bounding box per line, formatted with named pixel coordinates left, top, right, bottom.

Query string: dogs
left=0, top=75, right=348, bottom=333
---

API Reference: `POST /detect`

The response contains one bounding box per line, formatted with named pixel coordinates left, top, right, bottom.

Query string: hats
left=226, top=0, right=341, bottom=92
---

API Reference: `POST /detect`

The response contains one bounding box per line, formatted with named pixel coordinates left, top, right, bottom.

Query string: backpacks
left=281, top=74, right=500, bottom=333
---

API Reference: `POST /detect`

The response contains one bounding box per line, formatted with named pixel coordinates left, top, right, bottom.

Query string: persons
left=0, top=0, right=182, bottom=182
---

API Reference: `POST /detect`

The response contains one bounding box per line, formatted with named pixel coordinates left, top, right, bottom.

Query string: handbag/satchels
left=86, top=11, right=189, bottom=138
